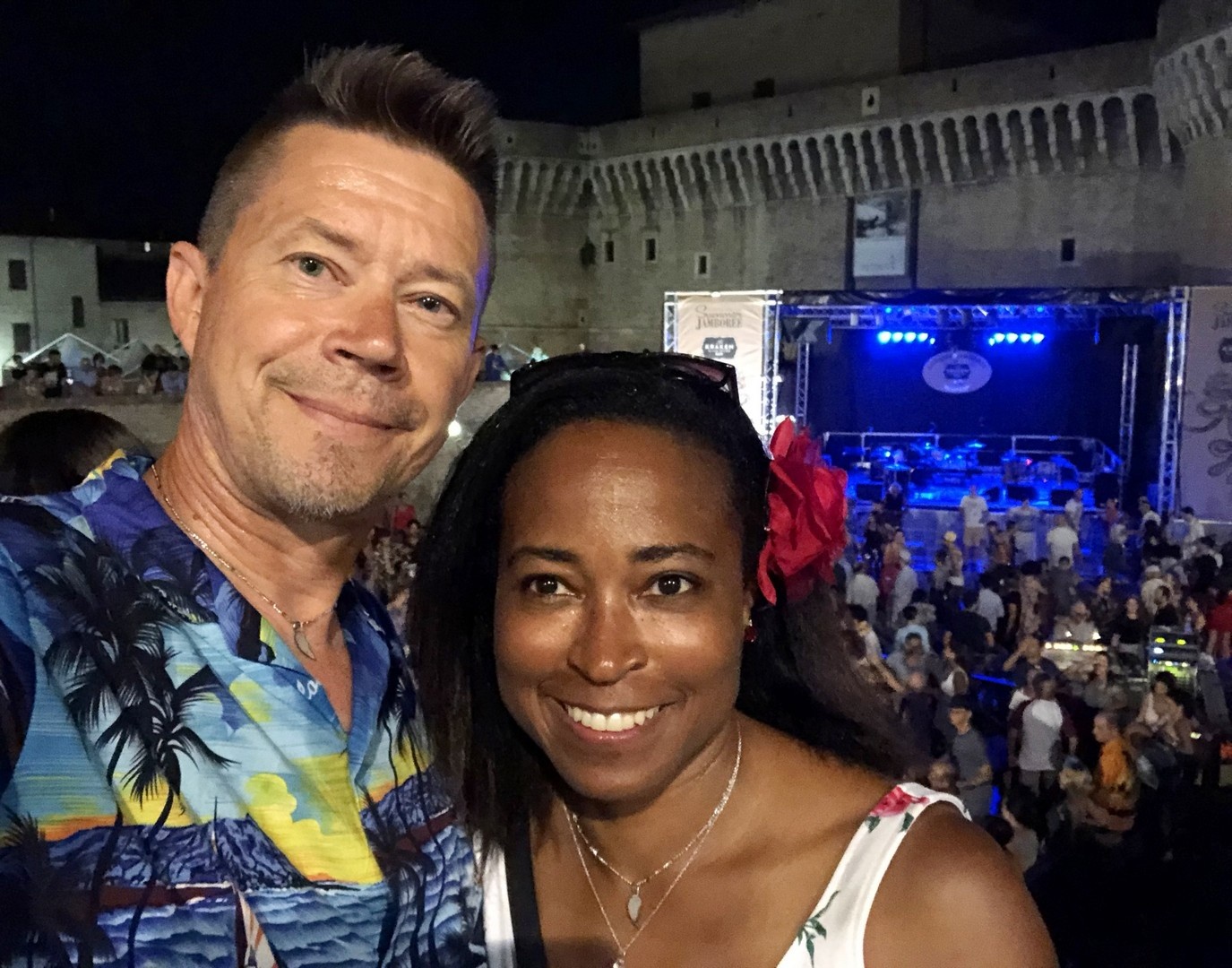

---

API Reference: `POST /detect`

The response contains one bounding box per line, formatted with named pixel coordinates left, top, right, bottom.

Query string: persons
left=832, top=482, right=1231, bottom=889
left=402, top=347, right=1060, bottom=968
left=0, top=37, right=514, bottom=968
left=1, top=341, right=510, bottom=638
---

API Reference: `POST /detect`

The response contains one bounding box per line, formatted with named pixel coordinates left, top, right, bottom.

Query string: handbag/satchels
left=1059, top=754, right=1092, bottom=791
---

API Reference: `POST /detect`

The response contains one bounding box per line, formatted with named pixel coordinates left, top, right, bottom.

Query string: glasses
left=510, top=351, right=739, bottom=409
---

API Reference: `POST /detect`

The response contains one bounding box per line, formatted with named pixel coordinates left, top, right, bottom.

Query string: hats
left=948, top=695, right=974, bottom=708
left=1071, top=602, right=1089, bottom=615
left=899, top=550, right=911, bottom=561
left=944, top=531, right=956, bottom=542
left=1145, top=565, right=1160, bottom=572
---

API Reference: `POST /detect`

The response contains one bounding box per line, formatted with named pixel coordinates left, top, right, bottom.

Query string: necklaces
left=561, top=718, right=742, bottom=968
left=150, top=463, right=338, bottom=659
